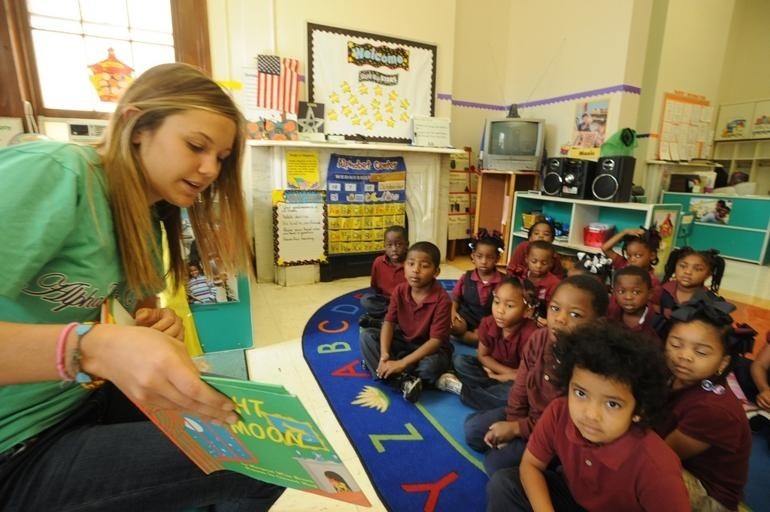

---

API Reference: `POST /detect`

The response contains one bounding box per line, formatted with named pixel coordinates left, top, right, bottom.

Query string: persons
left=650, top=245, right=726, bottom=321
left=360, top=224, right=408, bottom=334
left=576, top=112, right=591, bottom=131
left=505, top=221, right=565, bottom=286
left=523, top=240, right=561, bottom=319
left=643, top=292, right=752, bottom=511
left=716, top=201, right=725, bottom=222
left=0, top=62, right=288, bottom=512
left=466, top=273, right=608, bottom=478
left=598, top=222, right=659, bottom=289
left=360, top=241, right=455, bottom=403
left=451, top=228, right=508, bottom=345
left=700, top=208, right=729, bottom=222
left=439, top=276, right=539, bottom=411
left=605, top=265, right=661, bottom=340
left=485, top=317, right=693, bottom=511
left=185, top=260, right=216, bottom=304
left=735, top=324, right=769, bottom=452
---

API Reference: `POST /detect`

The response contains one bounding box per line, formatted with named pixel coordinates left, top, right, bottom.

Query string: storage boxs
left=581, top=223, right=615, bottom=248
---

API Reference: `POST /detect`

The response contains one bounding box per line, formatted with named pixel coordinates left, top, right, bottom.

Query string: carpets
left=300, top=277, right=524, bottom=511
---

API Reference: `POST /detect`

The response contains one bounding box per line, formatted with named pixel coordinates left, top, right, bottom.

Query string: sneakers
left=435, top=372, right=464, bottom=397
left=401, top=374, right=423, bottom=403
left=357, top=313, right=380, bottom=328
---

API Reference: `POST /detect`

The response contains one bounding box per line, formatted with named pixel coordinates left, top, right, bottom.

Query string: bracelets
left=69, top=321, right=108, bottom=389
left=58, top=321, right=80, bottom=383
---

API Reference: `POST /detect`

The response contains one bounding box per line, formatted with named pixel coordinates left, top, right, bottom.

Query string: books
left=99, top=293, right=371, bottom=507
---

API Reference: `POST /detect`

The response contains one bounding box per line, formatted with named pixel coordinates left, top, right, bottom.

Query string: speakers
left=541, top=157, right=566, bottom=198
left=589, top=156, right=636, bottom=202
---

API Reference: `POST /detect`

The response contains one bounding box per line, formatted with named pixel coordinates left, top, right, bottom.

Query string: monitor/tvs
left=483, top=118, right=545, bottom=171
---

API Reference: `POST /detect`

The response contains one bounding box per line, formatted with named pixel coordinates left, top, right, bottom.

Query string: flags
left=257, top=54, right=299, bottom=115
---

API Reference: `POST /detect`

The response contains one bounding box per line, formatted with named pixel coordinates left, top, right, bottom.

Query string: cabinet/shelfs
left=711, top=138, right=769, bottom=197
left=653, top=192, right=770, bottom=266
left=470, top=169, right=541, bottom=269
left=507, top=191, right=682, bottom=290
left=187, top=234, right=255, bottom=355
